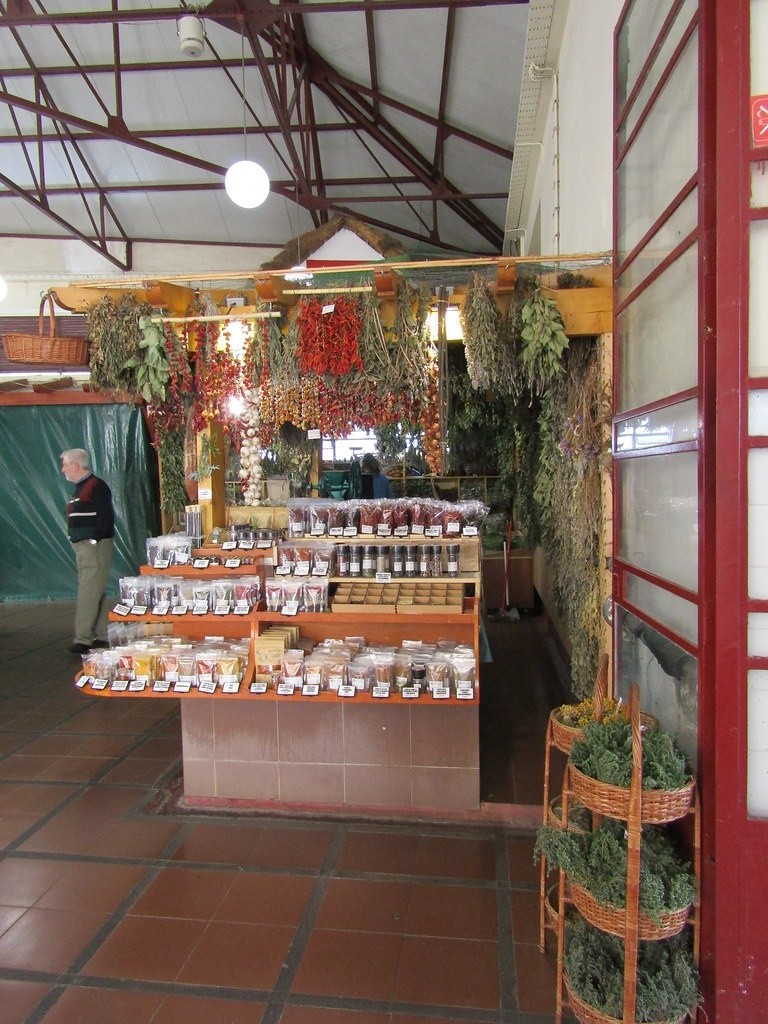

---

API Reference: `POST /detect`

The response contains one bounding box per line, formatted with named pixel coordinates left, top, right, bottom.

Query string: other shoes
left=69, top=643, right=92, bottom=654
left=91, top=638, right=109, bottom=648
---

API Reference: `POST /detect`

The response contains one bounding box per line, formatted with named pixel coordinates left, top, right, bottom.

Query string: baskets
left=1, top=294, right=90, bottom=365
left=545, top=883, right=572, bottom=939
left=567, top=759, right=697, bottom=824
left=551, top=702, right=660, bottom=756
left=548, top=793, right=589, bottom=836
left=563, top=972, right=688, bottom=1024
left=568, top=874, right=692, bottom=941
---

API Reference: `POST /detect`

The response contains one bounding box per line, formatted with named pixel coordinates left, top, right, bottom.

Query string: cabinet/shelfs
left=77, top=538, right=483, bottom=704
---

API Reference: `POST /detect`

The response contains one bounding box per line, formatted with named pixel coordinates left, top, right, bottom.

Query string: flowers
left=560, top=696, right=627, bottom=729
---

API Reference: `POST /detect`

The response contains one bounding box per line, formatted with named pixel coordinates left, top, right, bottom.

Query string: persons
left=60, top=449, right=115, bottom=652
left=349, top=453, right=393, bottom=499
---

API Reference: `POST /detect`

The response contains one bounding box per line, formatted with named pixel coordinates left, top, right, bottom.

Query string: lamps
left=226, top=11, right=270, bottom=208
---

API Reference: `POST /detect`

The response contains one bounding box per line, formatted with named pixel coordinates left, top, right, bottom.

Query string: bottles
left=336, top=544, right=460, bottom=578
